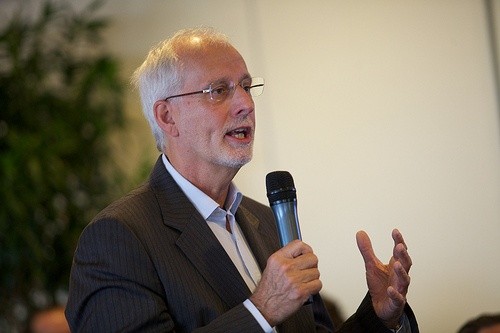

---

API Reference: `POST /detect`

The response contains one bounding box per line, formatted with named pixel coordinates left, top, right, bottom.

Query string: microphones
left=266, top=171, right=314, bottom=306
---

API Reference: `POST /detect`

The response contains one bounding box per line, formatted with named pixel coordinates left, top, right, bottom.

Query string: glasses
left=164, top=76, right=265, bottom=103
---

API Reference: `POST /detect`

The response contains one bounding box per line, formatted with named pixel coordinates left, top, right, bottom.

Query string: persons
left=64, top=26, right=422, bottom=332
left=457, top=314, right=499, bottom=333
left=28, top=306, right=73, bottom=332
